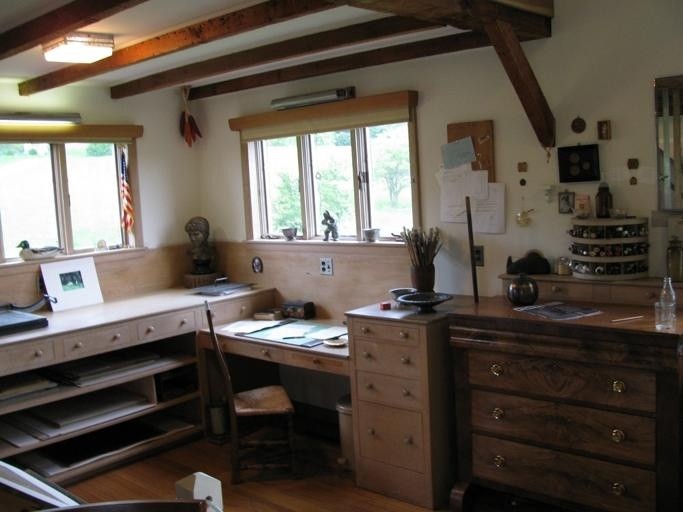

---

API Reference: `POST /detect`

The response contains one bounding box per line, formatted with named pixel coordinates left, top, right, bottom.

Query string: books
left=513, top=301, right=602, bottom=321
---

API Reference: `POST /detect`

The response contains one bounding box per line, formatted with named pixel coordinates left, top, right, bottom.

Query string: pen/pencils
left=282, top=336, right=304, bottom=339
left=611, top=316, right=644, bottom=323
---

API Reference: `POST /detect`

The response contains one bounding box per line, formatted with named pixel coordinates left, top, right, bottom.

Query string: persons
left=184, top=216, right=213, bottom=275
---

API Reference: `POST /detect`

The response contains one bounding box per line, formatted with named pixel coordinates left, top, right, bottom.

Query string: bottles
left=659, top=278, right=676, bottom=329
left=558, top=258, right=569, bottom=276
left=666, top=238, right=683, bottom=282
left=596, top=181, right=612, bottom=218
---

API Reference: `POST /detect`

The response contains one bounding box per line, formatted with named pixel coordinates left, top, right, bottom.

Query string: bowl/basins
left=387, top=287, right=418, bottom=305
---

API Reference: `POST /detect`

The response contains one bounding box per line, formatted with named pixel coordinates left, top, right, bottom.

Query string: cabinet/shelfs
left=343, top=292, right=493, bottom=511
left=0, top=284, right=276, bottom=484
left=446, top=273, right=683, bottom=512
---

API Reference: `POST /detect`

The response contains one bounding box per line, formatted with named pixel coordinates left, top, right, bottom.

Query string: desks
left=200, top=317, right=349, bottom=438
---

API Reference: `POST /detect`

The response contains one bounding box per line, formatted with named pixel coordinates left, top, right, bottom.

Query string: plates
left=397, top=292, right=454, bottom=313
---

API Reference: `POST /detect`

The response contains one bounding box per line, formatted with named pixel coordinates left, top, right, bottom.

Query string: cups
left=654, top=301, right=676, bottom=333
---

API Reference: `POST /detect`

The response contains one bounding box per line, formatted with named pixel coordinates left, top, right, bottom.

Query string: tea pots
left=507, top=273, right=539, bottom=307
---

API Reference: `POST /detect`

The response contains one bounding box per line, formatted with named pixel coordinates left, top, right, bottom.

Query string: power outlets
left=320, top=258, right=333, bottom=275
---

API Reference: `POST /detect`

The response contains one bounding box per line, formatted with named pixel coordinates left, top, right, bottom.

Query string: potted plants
left=391, top=226, right=446, bottom=292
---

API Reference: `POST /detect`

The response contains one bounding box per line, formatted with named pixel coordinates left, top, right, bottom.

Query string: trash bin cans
left=336, top=392, right=354, bottom=462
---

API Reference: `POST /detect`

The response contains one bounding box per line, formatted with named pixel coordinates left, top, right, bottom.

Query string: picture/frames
left=559, top=190, right=576, bottom=214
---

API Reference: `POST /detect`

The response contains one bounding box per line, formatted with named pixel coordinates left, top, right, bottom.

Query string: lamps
left=42, top=31, right=114, bottom=64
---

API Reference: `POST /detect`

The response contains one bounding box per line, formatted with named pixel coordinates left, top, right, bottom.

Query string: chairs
left=35, top=500, right=207, bottom=512
left=204, top=300, right=299, bottom=485
